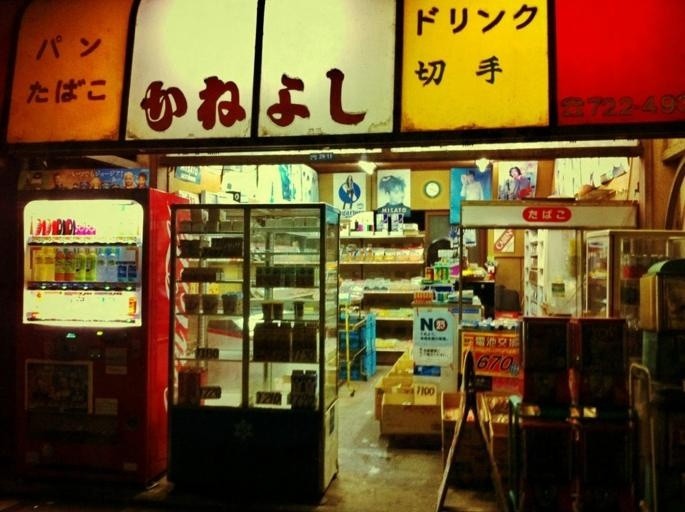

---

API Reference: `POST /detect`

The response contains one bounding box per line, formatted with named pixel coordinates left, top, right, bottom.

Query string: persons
left=508, top=167, right=530, bottom=199
left=124, top=172, right=137, bottom=189
left=71, top=183, right=78, bottom=190
left=375, top=176, right=411, bottom=217
left=51, top=173, right=68, bottom=190
left=343, top=174, right=354, bottom=209
left=135, top=172, right=148, bottom=188
left=460, top=170, right=483, bottom=201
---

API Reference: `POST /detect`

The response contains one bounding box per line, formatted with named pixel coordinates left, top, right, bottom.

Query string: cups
left=272, top=301, right=284, bottom=320
left=294, top=300, right=304, bottom=320
left=262, top=301, right=273, bottom=322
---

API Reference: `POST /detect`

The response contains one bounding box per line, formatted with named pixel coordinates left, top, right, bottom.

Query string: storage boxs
left=441, top=391, right=489, bottom=449
left=339, top=311, right=376, bottom=356
left=380, top=392, right=441, bottom=434
left=339, top=351, right=376, bottom=381
left=375, top=376, right=413, bottom=419
left=442, top=448, right=488, bottom=488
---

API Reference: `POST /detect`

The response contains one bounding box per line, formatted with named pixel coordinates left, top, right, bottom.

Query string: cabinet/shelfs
left=167, top=203, right=341, bottom=505
left=586, top=230, right=685, bottom=356
left=524, top=229, right=576, bottom=318
left=339, top=234, right=425, bottom=365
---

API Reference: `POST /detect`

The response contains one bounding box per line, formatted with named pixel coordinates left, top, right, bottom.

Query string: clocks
left=424, top=181, right=441, bottom=198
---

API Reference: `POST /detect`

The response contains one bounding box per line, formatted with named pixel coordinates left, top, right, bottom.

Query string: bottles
left=265, top=216, right=318, bottom=227
left=304, top=326, right=316, bottom=362
left=291, top=370, right=305, bottom=408
left=292, top=322, right=305, bottom=362
left=552, top=283, right=565, bottom=297
left=253, top=322, right=269, bottom=362
left=279, top=322, right=292, bottom=363
left=305, top=370, right=316, bottom=410
left=623, top=236, right=664, bottom=279
left=273, top=302, right=283, bottom=320
left=261, top=302, right=272, bottom=320
left=187, top=372, right=200, bottom=406
left=178, top=370, right=188, bottom=405
left=255, top=266, right=314, bottom=287
left=212, top=348, right=219, bottom=359
left=179, top=236, right=245, bottom=258
left=293, top=301, right=304, bottom=317
left=206, top=348, right=212, bottom=359
left=437, top=291, right=444, bottom=303
left=195, top=348, right=206, bottom=360
left=424, top=262, right=450, bottom=283
left=128, top=295, right=138, bottom=316
left=268, top=323, right=280, bottom=362
left=445, top=291, right=451, bottom=301
left=181, top=267, right=225, bottom=282
left=180, top=206, right=246, bottom=232
left=33, top=244, right=138, bottom=284
